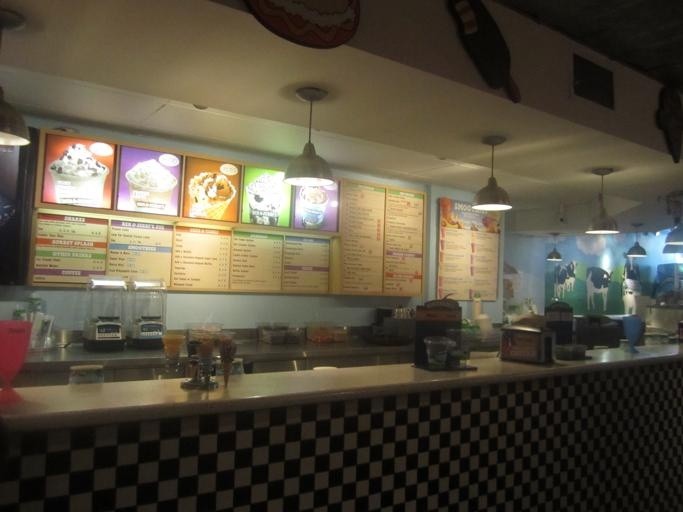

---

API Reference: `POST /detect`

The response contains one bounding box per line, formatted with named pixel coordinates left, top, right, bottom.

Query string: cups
left=126, top=171, right=177, bottom=214
left=246, top=187, right=286, bottom=226
left=187, top=176, right=237, bottom=221
left=49, top=162, right=109, bottom=208
left=423, top=336, right=451, bottom=370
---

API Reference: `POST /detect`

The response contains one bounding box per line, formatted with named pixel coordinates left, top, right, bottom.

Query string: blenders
left=27, top=294, right=57, bottom=352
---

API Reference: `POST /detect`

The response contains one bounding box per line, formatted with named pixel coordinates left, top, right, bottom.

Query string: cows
left=585, top=265, right=614, bottom=313
left=553, top=260, right=577, bottom=300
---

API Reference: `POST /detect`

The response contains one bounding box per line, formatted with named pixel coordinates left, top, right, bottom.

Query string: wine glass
left=0, top=320, right=33, bottom=407
left=622, top=316, right=641, bottom=354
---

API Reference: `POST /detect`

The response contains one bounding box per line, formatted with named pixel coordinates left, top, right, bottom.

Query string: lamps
left=283, top=85, right=336, bottom=187
left=546, top=168, right=650, bottom=262
left=472, top=135, right=514, bottom=213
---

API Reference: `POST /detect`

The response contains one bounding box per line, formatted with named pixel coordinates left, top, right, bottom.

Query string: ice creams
left=126, top=160, right=177, bottom=213
left=190, top=171, right=235, bottom=220
left=247, top=175, right=282, bottom=226
left=49, top=144, right=109, bottom=207
